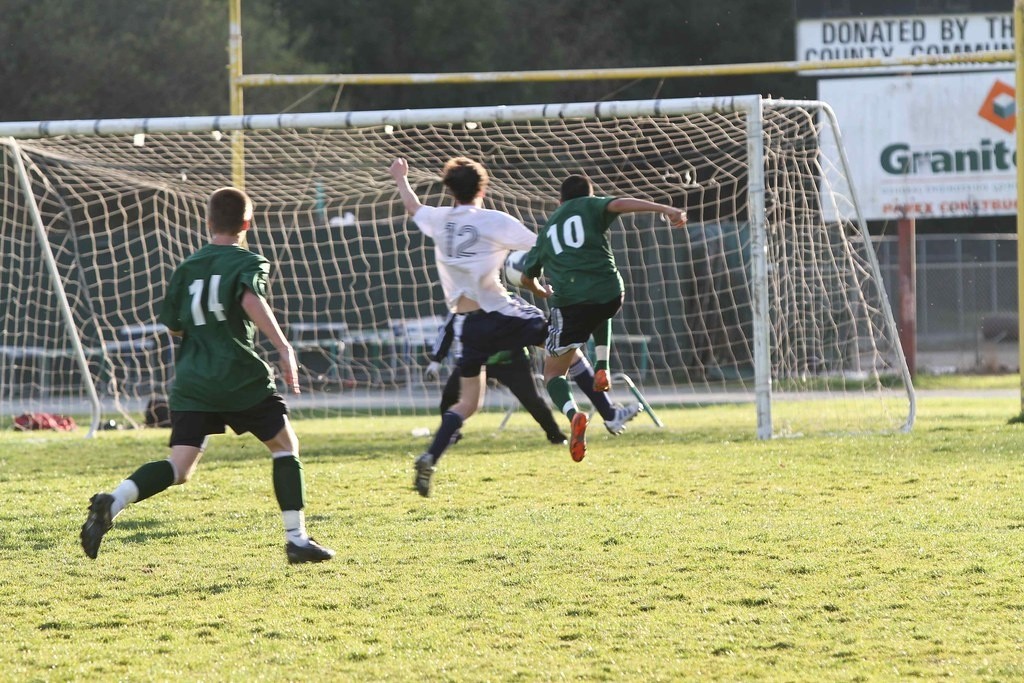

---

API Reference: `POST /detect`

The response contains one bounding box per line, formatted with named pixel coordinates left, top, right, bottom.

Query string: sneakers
left=592, top=369, right=610, bottom=393
left=287, top=541, right=334, bottom=563
left=603, top=402, right=643, bottom=436
left=79, top=492, right=114, bottom=559
left=569, top=413, right=588, bottom=462
left=414, top=454, right=436, bottom=496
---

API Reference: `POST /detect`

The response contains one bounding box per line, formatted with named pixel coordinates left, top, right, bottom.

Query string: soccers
left=505, top=250, right=544, bottom=288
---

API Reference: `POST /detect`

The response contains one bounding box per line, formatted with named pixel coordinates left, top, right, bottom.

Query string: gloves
left=426, top=361, right=441, bottom=381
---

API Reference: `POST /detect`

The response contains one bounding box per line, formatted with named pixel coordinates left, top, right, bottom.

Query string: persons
left=79, top=186, right=334, bottom=564
left=390, top=157, right=644, bottom=496
left=424, top=311, right=567, bottom=446
left=519, top=173, right=687, bottom=462
